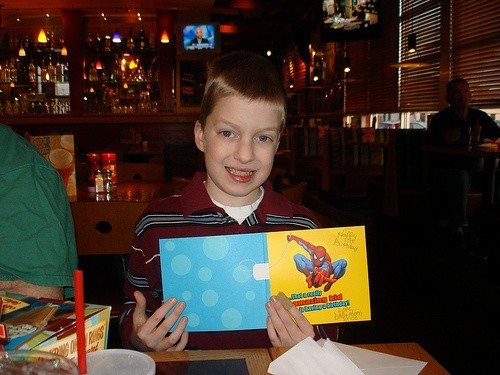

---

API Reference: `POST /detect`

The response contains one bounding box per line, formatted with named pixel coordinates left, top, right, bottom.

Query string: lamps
left=19, top=9, right=169, bottom=69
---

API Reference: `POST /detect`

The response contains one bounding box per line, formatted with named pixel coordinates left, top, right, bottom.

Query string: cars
left=380, top=121, right=425, bottom=130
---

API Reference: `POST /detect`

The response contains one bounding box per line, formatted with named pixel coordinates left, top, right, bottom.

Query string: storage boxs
left=0, top=291, right=112, bottom=362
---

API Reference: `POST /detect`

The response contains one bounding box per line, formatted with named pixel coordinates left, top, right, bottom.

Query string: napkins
left=268, top=336, right=428, bottom=375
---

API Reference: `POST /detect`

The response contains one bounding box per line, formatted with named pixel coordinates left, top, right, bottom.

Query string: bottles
left=86, top=152, right=118, bottom=195
left=0, top=25, right=158, bottom=104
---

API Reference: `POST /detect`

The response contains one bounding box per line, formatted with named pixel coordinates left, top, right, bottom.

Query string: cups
left=0, top=350, right=80, bottom=375
left=467, top=126, right=482, bottom=149
left=70, top=348, right=156, bottom=375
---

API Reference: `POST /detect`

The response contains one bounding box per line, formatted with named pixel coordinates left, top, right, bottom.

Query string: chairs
left=70, top=201, right=148, bottom=281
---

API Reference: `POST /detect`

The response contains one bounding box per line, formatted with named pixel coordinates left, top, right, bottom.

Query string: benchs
left=289, top=125, right=430, bottom=217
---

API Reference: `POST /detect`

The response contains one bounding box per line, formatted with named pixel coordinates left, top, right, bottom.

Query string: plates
left=478, top=145, right=498, bottom=148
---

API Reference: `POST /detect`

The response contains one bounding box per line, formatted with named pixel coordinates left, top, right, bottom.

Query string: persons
left=118, top=51, right=339, bottom=353
left=426, top=78, right=500, bottom=235
left=0, top=122, right=78, bottom=299
left=191, top=28, right=209, bottom=44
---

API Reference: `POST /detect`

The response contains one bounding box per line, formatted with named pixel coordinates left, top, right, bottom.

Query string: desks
left=144, top=342, right=452, bottom=375
left=75, top=161, right=165, bottom=200
left=450, top=149, right=500, bottom=203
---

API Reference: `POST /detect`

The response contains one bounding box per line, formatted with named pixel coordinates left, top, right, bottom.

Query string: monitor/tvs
left=179, top=22, right=219, bottom=54
